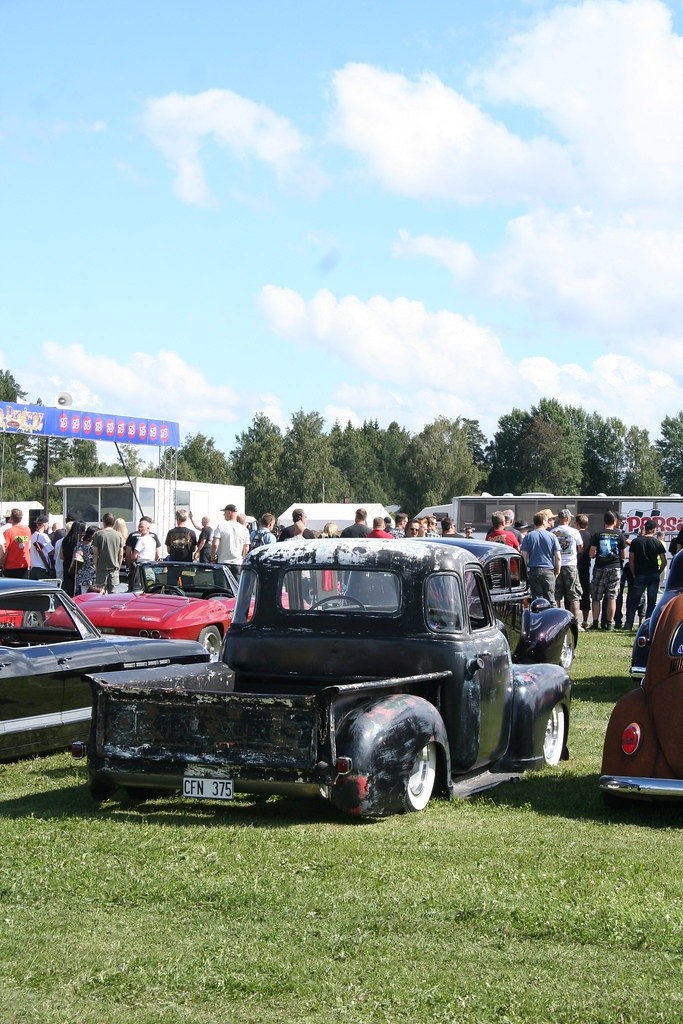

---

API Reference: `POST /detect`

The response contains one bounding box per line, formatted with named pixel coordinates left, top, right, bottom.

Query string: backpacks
left=248, top=530, right=269, bottom=552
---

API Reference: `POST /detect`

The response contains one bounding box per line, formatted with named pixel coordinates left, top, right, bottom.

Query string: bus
left=451, top=491, right=683, bottom=589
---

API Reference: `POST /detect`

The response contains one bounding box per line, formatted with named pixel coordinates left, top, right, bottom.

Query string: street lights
left=36, top=392, right=72, bottom=517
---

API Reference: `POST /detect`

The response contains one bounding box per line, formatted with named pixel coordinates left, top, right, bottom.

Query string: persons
left=0, top=508, right=162, bottom=597
left=486, top=508, right=683, bottom=631
left=165, top=509, right=198, bottom=585
left=189, top=511, right=214, bottom=563
left=212, top=504, right=474, bottom=610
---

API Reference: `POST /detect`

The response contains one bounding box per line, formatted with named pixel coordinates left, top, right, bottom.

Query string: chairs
left=200, top=587, right=233, bottom=600
left=150, top=585, right=185, bottom=596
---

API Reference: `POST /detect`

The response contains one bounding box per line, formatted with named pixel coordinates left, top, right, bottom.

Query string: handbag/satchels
left=68, top=546, right=85, bottom=576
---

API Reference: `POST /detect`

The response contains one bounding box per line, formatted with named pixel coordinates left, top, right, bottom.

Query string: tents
left=277, top=502, right=395, bottom=532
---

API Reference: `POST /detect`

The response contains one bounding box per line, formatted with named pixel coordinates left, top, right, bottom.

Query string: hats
left=384, top=517, right=391, bottom=524
left=615, top=511, right=627, bottom=520
left=513, top=520, right=529, bottom=529
left=559, top=509, right=574, bottom=518
left=36, top=515, right=48, bottom=527
left=220, top=504, right=237, bottom=512
left=3, top=509, right=12, bottom=518
left=539, top=509, right=557, bottom=519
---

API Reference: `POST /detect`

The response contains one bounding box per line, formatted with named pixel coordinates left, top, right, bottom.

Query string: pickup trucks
left=71, top=537, right=580, bottom=818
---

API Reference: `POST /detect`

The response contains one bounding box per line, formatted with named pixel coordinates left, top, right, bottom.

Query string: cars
left=321, top=535, right=579, bottom=668
left=0, top=578, right=213, bottom=764
left=597, top=591, right=683, bottom=810
left=629, top=548, right=683, bottom=681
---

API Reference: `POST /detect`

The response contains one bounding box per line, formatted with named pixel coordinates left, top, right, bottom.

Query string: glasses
left=303, top=515, right=307, bottom=518
left=549, top=518, right=556, bottom=522
left=428, top=522, right=434, bottom=526
left=436, top=523, right=438, bottom=526
left=452, top=525, right=456, bottom=528
left=408, top=528, right=419, bottom=531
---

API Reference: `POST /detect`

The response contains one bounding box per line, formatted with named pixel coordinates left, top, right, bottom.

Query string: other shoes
left=577, top=620, right=632, bottom=631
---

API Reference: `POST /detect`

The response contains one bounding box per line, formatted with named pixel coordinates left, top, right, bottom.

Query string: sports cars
left=41, top=559, right=313, bottom=663
